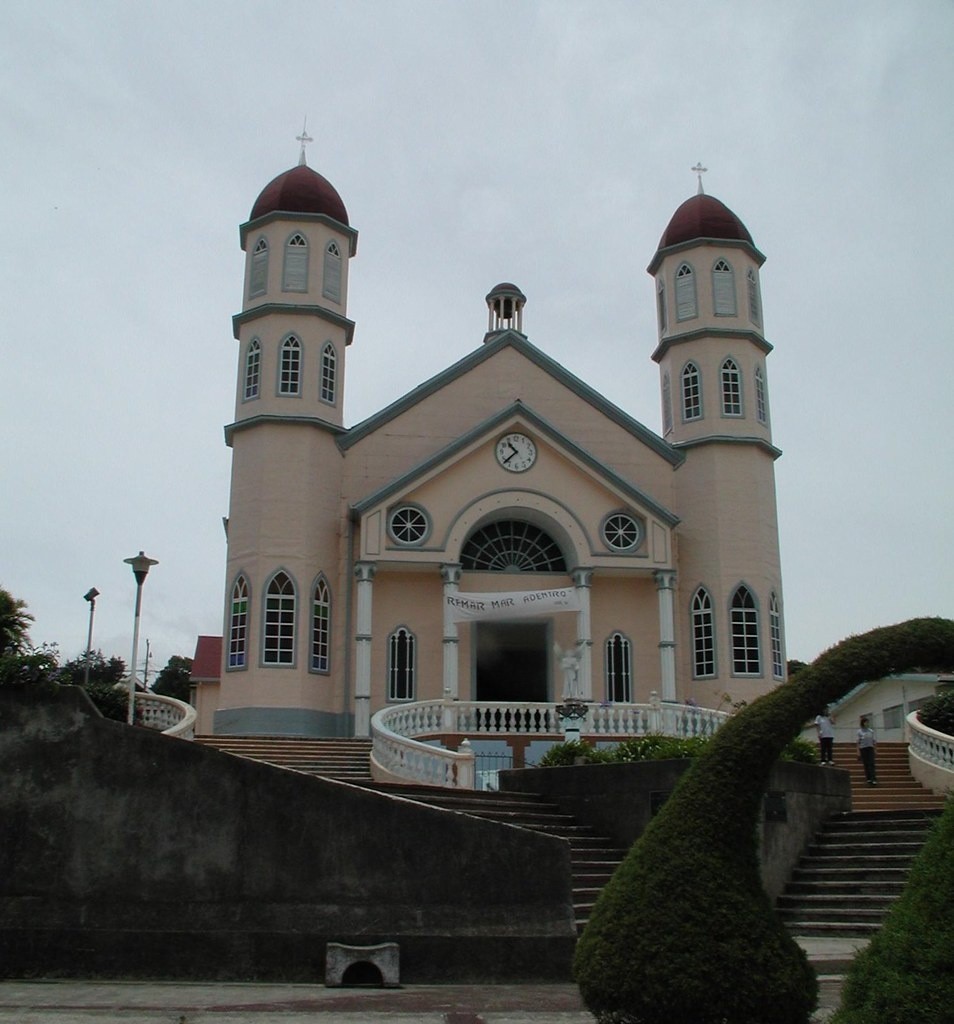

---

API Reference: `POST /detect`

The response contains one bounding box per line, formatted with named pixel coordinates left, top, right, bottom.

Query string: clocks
left=495, top=431, right=537, bottom=473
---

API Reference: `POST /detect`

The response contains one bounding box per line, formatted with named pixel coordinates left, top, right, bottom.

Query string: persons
left=812, top=703, right=839, bottom=765
left=857, top=717, right=877, bottom=784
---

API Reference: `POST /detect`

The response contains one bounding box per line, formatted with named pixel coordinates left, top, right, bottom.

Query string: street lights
left=124, top=550, right=160, bottom=725
left=81, top=587, right=102, bottom=694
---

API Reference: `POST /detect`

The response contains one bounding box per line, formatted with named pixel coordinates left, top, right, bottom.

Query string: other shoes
left=867, top=779, right=871, bottom=782
left=821, top=762, right=826, bottom=765
left=828, top=760, right=835, bottom=765
left=872, top=781, right=877, bottom=784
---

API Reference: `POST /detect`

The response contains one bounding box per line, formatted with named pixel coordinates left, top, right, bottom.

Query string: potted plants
left=567, top=740, right=592, bottom=766
